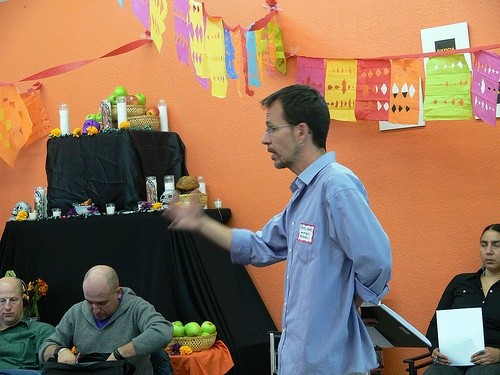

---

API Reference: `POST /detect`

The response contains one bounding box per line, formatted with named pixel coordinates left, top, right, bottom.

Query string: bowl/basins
left=75, top=206, right=90, bottom=214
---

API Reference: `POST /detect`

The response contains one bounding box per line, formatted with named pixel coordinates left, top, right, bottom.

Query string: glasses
left=266, top=124, right=311, bottom=136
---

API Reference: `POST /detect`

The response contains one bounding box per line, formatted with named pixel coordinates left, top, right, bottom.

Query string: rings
left=434, top=356, right=440, bottom=365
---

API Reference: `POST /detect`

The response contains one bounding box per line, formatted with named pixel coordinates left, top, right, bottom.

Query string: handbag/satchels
left=39, top=352, right=132, bottom=375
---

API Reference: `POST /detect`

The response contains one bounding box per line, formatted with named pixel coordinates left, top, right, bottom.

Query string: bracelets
left=112, top=348, right=128, bottom=361
left=53, top=346, right=70, bottom=362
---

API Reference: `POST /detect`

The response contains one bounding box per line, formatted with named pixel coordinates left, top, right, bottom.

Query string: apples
left=108, top=86, right=146, bottom=106
left=171, top=321, right=216, bottom=338
left=145, top=107, right=159, bottom=116
left=85, top=113, right=103, bottom=123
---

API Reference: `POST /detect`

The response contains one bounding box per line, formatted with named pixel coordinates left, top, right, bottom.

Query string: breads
left=176, top=176, right=199, bottom=190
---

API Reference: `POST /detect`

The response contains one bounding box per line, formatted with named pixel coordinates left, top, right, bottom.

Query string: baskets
left=171, top=333, right=217, bottom=351
left=127, top=115, right=160, bottom=129
left=179, top=193, right=207, bottom=209
left=109, top=104, right=146, bottom=120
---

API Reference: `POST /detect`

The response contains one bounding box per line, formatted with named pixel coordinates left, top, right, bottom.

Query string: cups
left=214, top=199, right=222, bottom=208
left=29, top=210, right=38, bottom=219
left=138, top=201, right=145, bottom=210
left=182, top=199, right=190, bottom=207
left=52, top=208, right=62, bottom=216
left=106, top=203, right=115, bottom=214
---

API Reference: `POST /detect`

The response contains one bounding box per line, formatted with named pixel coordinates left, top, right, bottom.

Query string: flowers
left=118, top=119, right=130, bottom=130
left=16, top=211, right=28, bottom=221
left=5, top=270, right=48, bottom=321
left=85, top=126, right=99, bottom=136
left=151, top=202, right=162, bottom=211
left=49, top=128, right=63, bottom=137
left=72, top=128, right=82, bottom=137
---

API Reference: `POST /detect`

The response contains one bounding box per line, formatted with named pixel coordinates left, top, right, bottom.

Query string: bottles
left=100, top=99, right=112, bottom=130
left=34, top=185, right=46, bottom=220
left=59, top=103, right=71, bottom=135
left=117, top=96, right=127, bottom=128
left=157, top=98, right=170, bottom=132
left=195, top=176, right=208, bottom=209
left=146, top=176, right=157, bottom=209
left=164, top=176, right=174, bottom=191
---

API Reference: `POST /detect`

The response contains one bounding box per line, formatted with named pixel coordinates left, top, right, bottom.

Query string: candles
left=59, top=109, right=69, bottom=135
left=116, top=103, right=126, bottom=122
left=29, top=212, right=37, bottom=220
left=214, top=200, right=222, bottom=208
left=53, top=211, right=61, bottom=217
left=106, top=206, right=114, bottom=214
left=158, top=105, right=169, bottom=131
left=199, top=182, right=208, bottom=209
left=164, top=182, right=174, bottom=191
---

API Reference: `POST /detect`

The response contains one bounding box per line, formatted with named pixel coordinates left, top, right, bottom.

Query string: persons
left=423, top=224, right=500, bottom=375
left=38, top=265, right=174, bottom=375
left=161, top=83, right=393, bottom=375
left=0, top=276, right=55, bottom=375
